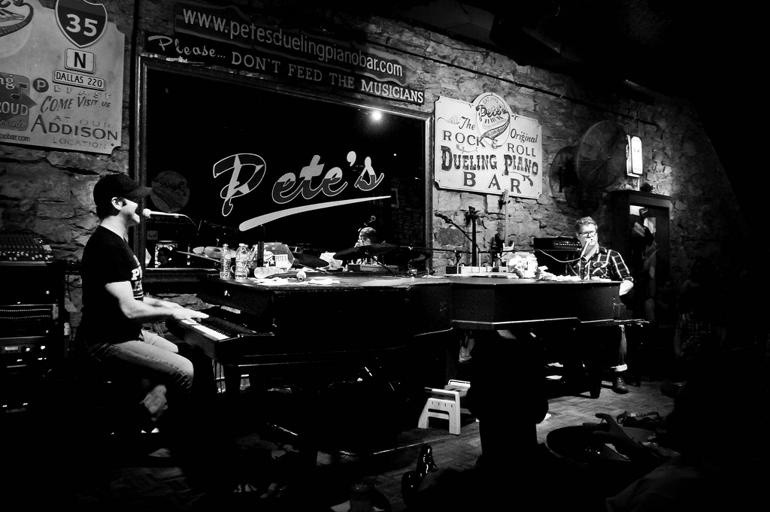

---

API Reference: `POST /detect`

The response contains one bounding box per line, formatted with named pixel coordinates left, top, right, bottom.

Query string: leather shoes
left=613, top=376, right=629, bottom=394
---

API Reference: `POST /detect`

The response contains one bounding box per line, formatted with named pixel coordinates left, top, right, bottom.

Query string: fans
left=560, top=120, right=628, bottom=190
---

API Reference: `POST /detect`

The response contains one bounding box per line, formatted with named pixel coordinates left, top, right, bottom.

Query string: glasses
left=580, top=231, right=597, bottom=238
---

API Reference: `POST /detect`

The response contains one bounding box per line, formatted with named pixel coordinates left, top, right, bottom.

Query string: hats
left=94, top=173, right=152, bottom=202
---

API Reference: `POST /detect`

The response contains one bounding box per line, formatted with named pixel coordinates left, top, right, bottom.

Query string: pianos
left=152, top=276, right=456, bottom=451
left=425, top=274, right=649, bottom=401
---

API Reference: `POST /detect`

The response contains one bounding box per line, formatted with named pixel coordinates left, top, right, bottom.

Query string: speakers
left=0, top=262, right=66, bottom=436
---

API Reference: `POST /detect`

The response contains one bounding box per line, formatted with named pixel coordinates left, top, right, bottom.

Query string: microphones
left=433, top=211, right=450, bottom=223
left=578, top=239, right=591, bottom=259
left=143, top=208, right=184, bottom=221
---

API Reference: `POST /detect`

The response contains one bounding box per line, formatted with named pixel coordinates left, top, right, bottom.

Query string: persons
left=76, top=173, right=196, bottom=457
left=564, top=216, right=635, bottom=394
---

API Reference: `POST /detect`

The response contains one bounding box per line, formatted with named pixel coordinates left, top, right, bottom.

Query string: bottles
left=235, top=242, right=249, bottom=284
left=220, top=242, right=232, bottom=282
left=254, top=266, right=284, bottom=278
